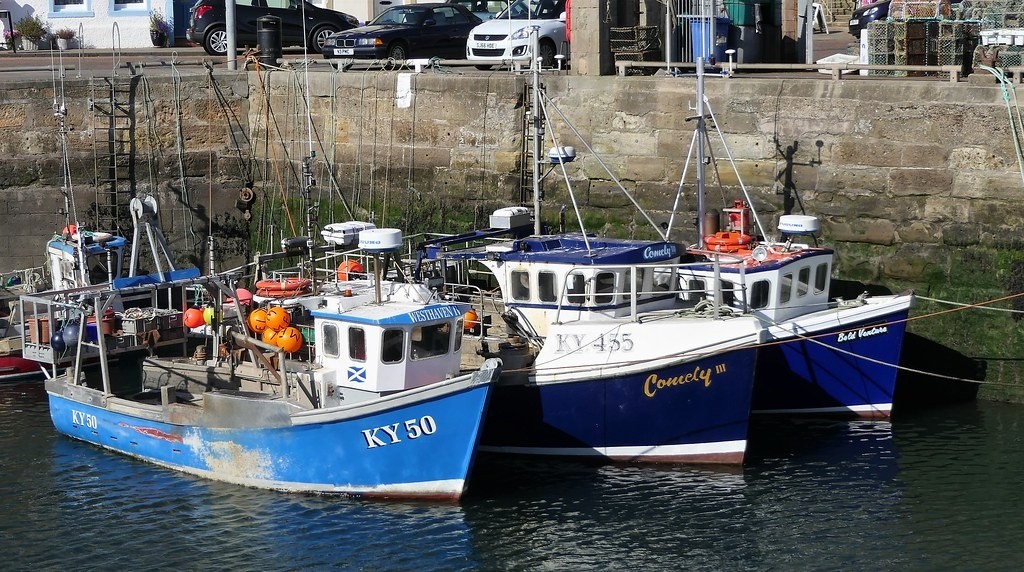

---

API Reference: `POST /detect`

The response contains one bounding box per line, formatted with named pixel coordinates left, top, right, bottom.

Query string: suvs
left=186, top=0, right=360, bottom=56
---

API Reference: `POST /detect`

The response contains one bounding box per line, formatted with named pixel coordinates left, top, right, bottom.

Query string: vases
left=6, top=38, right=12, bottom=49
left=150, top=30, right=165, bottom=46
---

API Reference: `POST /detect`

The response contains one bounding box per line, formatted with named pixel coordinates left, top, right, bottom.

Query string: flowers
left=148, top=8, right=170, bottom=35
left=4, top=31, right=20, bottom=39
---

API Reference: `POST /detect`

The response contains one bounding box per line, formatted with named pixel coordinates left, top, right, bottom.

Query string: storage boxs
left=980, top=28, right=1024, bottom=46
left=116, top=311, right=185, bottom=334
left=816, top=53, right=858, bottom=75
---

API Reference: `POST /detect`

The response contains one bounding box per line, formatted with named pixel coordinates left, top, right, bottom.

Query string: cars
left=441, top=0, right=535, bottom=31
left=847, top=0, right=892, bottom=40
left=465, top=0, right=569, bottom=71
left=321, top=3, right=485, bottom=71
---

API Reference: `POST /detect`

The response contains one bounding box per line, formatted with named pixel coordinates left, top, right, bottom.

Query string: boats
left=0, top=0, right=916, bottom=509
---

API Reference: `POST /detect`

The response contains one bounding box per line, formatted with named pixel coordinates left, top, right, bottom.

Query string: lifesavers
left=704, top=231, right=752, bottom=246
left=707, top=244, right=750, bottom=253
left=256, top=277, right=311, bottom=291
left=256, top=289, right=308, bottom=298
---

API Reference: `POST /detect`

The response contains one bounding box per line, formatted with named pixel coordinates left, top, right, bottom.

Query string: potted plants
left=55, top=28, right=75, bottom=50
left=16, top=12, right=48, bottom=51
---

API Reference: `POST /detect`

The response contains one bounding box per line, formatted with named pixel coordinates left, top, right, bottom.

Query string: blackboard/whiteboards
left=813, top=3, right=827, bottom=29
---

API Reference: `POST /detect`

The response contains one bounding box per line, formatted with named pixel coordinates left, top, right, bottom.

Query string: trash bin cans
left=257, top=15, right=282, bottom=69
left=689, top=17, right=729, bottom=73
